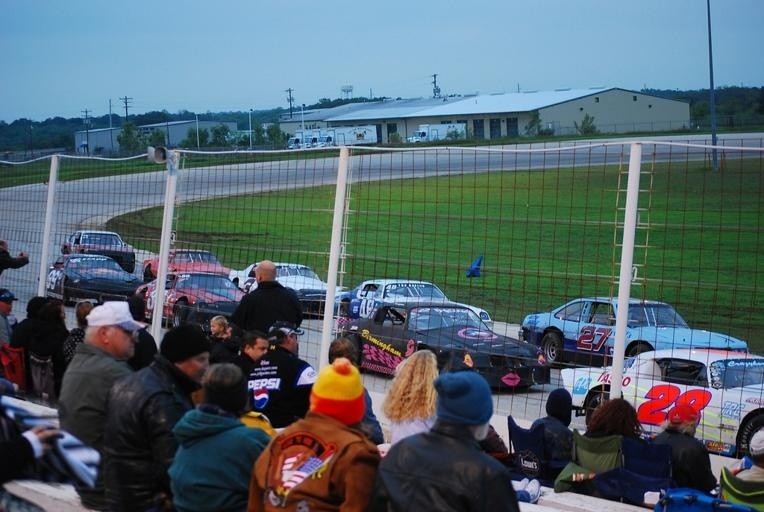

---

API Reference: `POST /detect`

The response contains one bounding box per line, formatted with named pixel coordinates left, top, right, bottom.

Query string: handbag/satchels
left=239, top=411, right=276, bottom=440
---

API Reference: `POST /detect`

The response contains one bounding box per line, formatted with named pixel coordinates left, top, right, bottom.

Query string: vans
left=317, top=136, right=332, bottom=147
left=287, top=135, right=300, bottom=149
left=305, top=136, right=317, bottom=148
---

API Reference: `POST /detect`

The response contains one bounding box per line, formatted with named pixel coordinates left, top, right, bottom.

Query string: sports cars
left=560, top=348, right=764, bottom=459
left=328, top=278, right=493, bottom=330
left=340, top=302, right=550, bottom=392
left=42, top=230, right=341, bottom=333
left=518, top=295, right=752, bottom=369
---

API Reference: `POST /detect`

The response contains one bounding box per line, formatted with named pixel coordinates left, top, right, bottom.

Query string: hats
left=668, top=404, right=702, bottom=426
left=265, top=318, right=304, bottom=345
left=85, top=301, right=149, bottom=332
left=0, top=289, right=18, bottom=302
left=750, top=428, right=764, bottom=456
left=309, top=357, right=365, bottom=426
left=160, top=322, right=213, bottom=364
left=432, top=369, right=493, bottom=428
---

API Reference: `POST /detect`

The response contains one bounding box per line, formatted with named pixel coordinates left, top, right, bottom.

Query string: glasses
left=110, top=326, right=140, bottom=338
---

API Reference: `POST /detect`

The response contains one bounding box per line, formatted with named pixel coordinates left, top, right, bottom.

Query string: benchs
left=4, top=479, right=98, bottom=512
left=3, top=396, right=59, bottom=428
left=510, top=481, right=653, bottom=512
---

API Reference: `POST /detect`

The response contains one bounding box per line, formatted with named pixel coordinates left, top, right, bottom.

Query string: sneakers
left=524, top=479, right=541, bottom=504
left=514, top=478, right=529, bottom=492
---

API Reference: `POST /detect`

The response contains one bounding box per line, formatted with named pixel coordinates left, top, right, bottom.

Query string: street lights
left=301, top=103, right=308, bottom=149
left=248, top=107, right=255, bottom=149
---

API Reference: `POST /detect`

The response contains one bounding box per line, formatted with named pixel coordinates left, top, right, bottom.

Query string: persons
left=128, top=294, right=158, bottom=368
left=64, top=300, right=93, bottom=358
left=583, top=398, right=666, bottom=499
left=246, top=320, right=317, bottom=430
left=58, top=300, right=148, bottom=449
left=528, top=388, right=573, bottom=459
left=736, top=427, right=764, bottom=482
left=1, top=287, right=17, bottom=344
left=0, top=423, right=65, bottom=478
left=382, top=350, right=438, bottom=445
left=98, top=323, right=210, bottom=512
left=247, top=357, right=380, bottom=512
left=329, top=335, right=384, bottom=444
left=210, top=315, right=230, bottom=338
left=235, top=331, right=270, bottom=376
left=652, top=405, right=717, bottom=493
left=0, top=239, right=30, bottom=277
left=167, top=362, right=271, bottom=511
left=11, top=297, right=45, bottom=344
left=231, top=260, right=303, bottom=336
left=33, top=299, right=68, bottom=351
left=369, top=370, right=522, bottom=512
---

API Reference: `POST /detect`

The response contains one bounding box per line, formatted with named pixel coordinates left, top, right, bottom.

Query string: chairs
left=718, top=465, right=764, bottom=512
left=507, top=415, right=571, bottom=488
left=622, top=437, right=677, bottom=512
left=572, top=428, right=624, bottom=472
